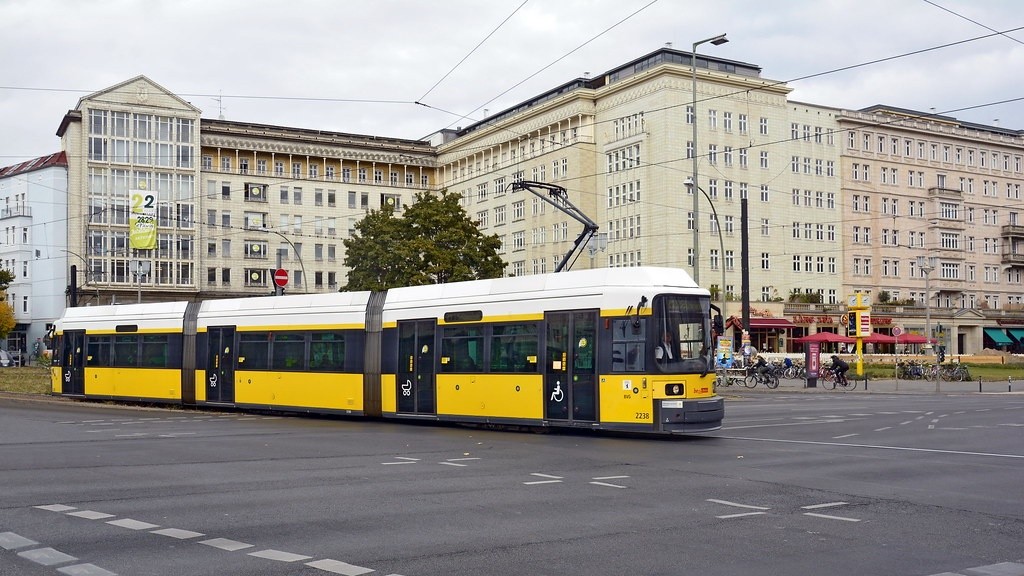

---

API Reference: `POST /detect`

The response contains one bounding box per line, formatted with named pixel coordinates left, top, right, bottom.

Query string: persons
left=655, top=331, right=674, bottom=364
left=829, top=355, right=849, bottom=386
left=783, top=358, right=792, bottom=368
left=319, top=354, right=333, bottom=370
left=753, top=355, right=771, bottom=384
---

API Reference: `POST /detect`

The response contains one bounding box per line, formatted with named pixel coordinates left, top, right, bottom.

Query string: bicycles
left=896, top=358, right=973, bottom=382
left=716, top=359, right=857, bottom=391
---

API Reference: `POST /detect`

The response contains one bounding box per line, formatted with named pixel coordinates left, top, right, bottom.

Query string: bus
left=44, top=181, right=725, bottom=437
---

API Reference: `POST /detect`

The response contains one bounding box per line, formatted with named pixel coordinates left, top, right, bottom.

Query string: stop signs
left=273, top=268, right=288, bottom=288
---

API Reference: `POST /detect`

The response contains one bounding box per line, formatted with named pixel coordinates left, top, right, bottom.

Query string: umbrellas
left=795, top=332, right=856, bottom=352
left=847, top=332, right=897, bottom=353
left=897, top=333, right=937, bottom=353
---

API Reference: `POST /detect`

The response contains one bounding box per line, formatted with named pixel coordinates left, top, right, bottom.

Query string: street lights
left=258, top=228, right=307, bottom=293
left=682, top=175, right=727, bottom=338
left=916, top=254, right=937, bottom=357
left=691, top=32, right=730, bottom=293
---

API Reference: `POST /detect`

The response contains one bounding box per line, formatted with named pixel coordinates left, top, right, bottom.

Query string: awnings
left=735, top=318, right=796, bottom=328
left=1007, top=328, right=1024, bottom=346
left=984, top=328, right=1014, bottom=346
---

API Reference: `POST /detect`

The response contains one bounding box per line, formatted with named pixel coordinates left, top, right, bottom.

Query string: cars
left=0, top=347, right=16, bottom=367
left=7, top=351, right=25, bottom=366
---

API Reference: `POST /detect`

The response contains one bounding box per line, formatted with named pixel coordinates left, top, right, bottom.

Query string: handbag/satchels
left=766, top=365, right=775, bottom=372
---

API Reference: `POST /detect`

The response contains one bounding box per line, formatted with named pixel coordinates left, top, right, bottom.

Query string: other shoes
left=757, top=378, right=762, bottom=382
left=844, top=382, right=847, bottom=386
left=835, top=380, right=841, bottom=383
left=767, top=379, right=770, bottom=383
